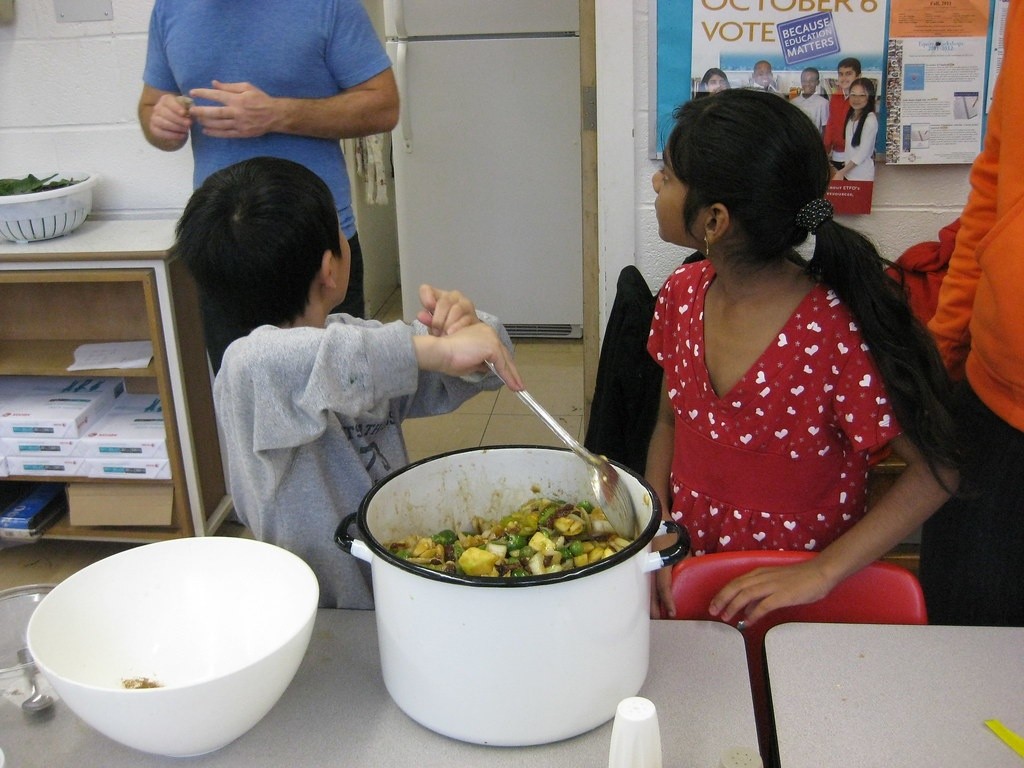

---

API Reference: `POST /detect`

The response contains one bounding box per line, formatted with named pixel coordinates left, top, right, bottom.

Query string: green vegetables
left=0, top=172, right=88, bottom=196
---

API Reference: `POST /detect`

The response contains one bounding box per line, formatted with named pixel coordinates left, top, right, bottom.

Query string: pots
left=336, top=446, right=689, bottom=747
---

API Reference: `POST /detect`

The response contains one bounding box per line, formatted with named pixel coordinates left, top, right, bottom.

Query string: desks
left=762, top=621, right=1024, bottom=768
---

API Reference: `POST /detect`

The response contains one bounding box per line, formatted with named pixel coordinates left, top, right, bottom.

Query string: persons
left=136, top=0, right=401, bottom=377
left=644, top=88, right=992, bottom=630
left=697, top=56, right=879, bottom=182
left=921, top=1, right=1024, bottom=627
left=176, top=155, right=528, bottom=609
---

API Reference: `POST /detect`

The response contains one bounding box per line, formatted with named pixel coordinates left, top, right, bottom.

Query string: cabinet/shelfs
left=0, top=217, right=233, bottom=545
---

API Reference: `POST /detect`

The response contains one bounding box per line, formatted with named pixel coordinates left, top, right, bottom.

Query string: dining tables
left=0, top=609, right=762, bottom=768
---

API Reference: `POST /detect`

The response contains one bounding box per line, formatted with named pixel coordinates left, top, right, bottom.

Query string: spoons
left=14, top=649, right=52, bottom=714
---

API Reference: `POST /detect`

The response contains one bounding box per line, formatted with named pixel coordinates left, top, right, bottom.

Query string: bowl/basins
left=0, top=584, right=70, bottom=713
left=28, top=537, right=318, bottom=756
